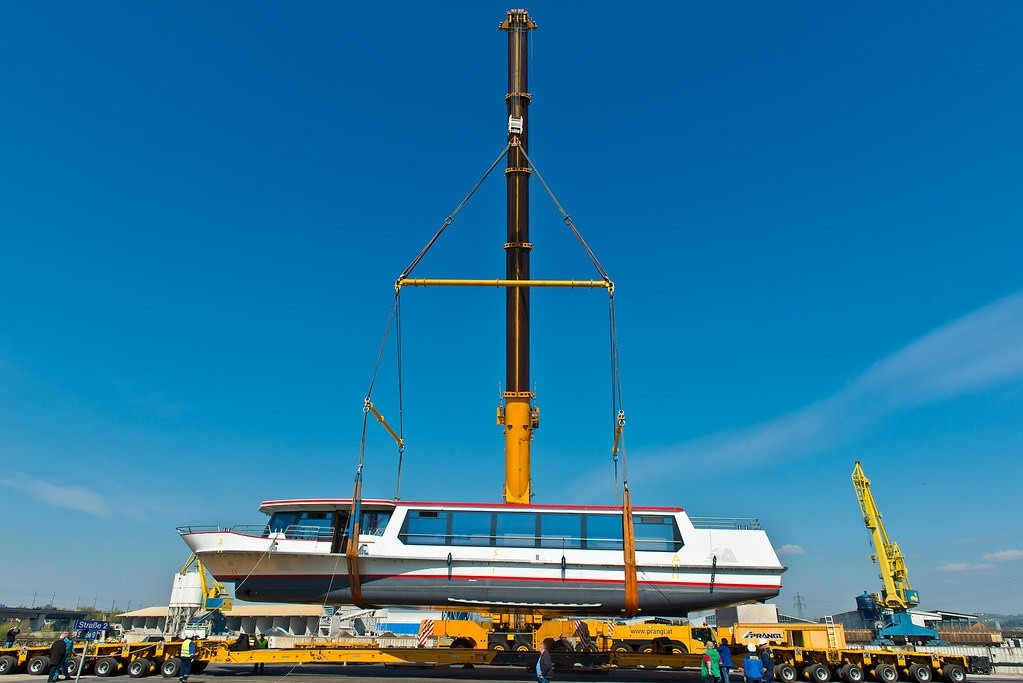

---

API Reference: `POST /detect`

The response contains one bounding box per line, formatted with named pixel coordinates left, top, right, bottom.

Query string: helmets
left=758, top=638, right=767, bottom=645
left=187, top=633, right=194, bottom=638
left=260, top=632, right=265, bottom=636
left=747, top=643, right=756, bottom=652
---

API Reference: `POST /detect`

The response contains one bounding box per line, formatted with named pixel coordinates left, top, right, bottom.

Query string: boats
left=175, top=495, right=787, bottom=611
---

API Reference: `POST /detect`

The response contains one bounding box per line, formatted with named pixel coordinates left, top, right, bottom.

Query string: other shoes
left=180, top=680, right=188, bottom=683
left=47, top=678, right=73, bottom=682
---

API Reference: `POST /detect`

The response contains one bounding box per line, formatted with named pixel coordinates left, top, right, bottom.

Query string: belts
left=538, top=675, right=544, bottom=678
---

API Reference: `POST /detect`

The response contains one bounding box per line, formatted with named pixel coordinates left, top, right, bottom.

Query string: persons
left=47, top=630, right=75, bottom=682
left=702, top=638, right=773, bottom=683
left=253, top=633, right=268, bottom=674
left=179, top=631, right=195, bottom=682
left=536, top=642, right=553, bottom=683
left=5, top=626, right=21, bottom=648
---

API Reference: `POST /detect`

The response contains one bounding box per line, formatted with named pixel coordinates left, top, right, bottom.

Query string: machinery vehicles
left=169, top=545, right=234, bottom=642
left=847, top=455, right=946, bottom=652
left=0, top=8, right=1006, bottom=683
